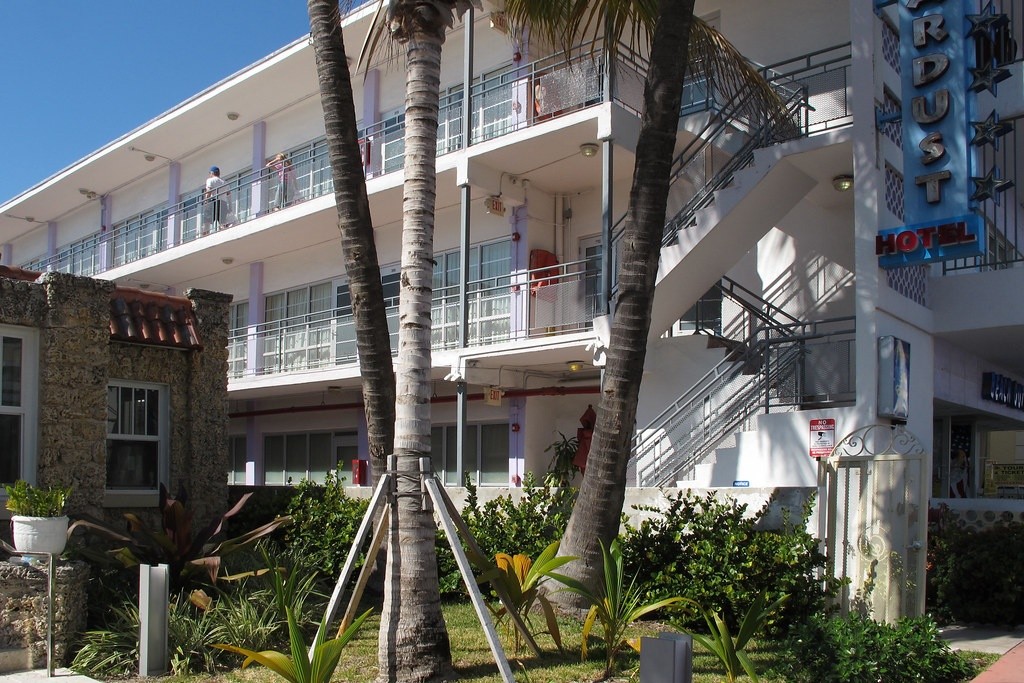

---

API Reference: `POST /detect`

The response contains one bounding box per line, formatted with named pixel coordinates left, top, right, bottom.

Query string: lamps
left=579, top=144, right=600, bottom=159
left=567, top=361, right=584, bottom=372
left=833, top=177, right=853, bottom=192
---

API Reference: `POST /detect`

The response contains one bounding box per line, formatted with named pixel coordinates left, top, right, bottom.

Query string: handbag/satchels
left=226, top=211, right=238, bottom=225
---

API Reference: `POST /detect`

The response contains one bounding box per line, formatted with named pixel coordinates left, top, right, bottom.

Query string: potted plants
left=5, top=480, right=76, bottom=561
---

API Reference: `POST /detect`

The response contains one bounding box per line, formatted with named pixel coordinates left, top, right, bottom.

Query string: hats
left=209, top=166, right=219, bottom=173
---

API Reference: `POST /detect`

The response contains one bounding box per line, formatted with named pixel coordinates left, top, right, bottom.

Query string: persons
left=265, top=153, right=298, bottom=213
left=199, top=165, right=233, bottom=236
